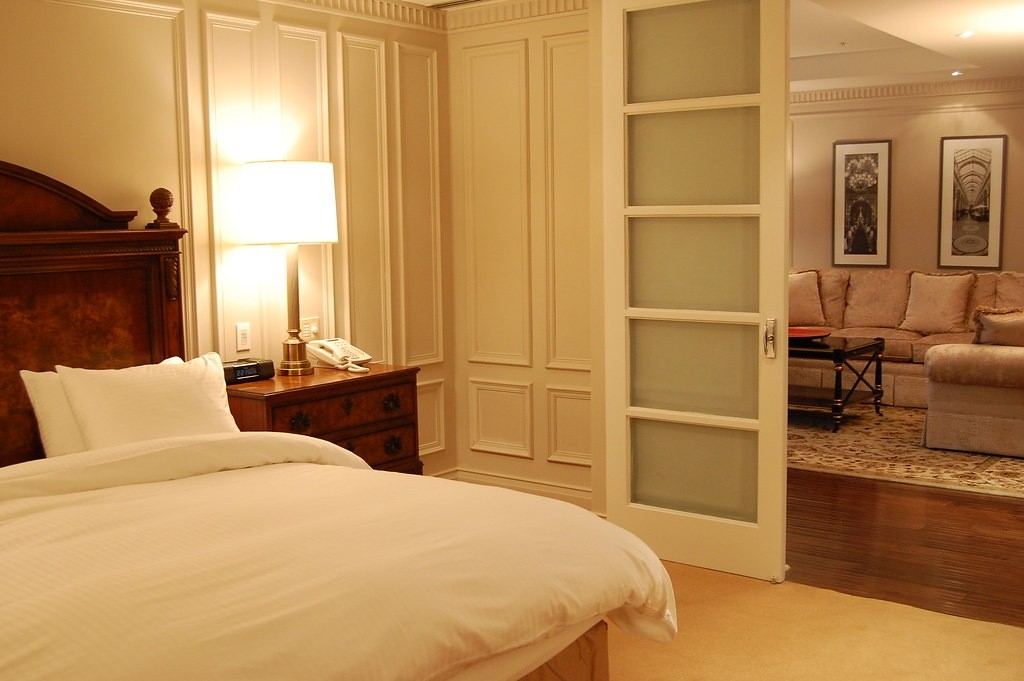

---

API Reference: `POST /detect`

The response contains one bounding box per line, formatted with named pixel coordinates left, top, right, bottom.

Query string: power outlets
left=300, top=317, right=318, bottom=344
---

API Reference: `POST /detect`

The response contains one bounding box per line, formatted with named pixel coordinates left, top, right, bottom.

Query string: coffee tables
left=788, top=337, right=885, bottom=433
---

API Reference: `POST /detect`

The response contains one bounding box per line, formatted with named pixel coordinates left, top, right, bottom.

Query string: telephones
left=306, top=337, right=372, bottom=370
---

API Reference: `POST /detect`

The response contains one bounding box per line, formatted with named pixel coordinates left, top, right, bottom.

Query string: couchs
left=788, top=269, right=1024, bottom=458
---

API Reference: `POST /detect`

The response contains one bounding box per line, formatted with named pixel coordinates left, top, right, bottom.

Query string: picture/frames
left=832, top=139, right=892, bottom=269
left=937, top=134, right=1007, bottom=271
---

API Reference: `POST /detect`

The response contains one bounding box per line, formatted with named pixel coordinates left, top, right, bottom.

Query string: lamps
left=222, top=162, right=338, bottom=377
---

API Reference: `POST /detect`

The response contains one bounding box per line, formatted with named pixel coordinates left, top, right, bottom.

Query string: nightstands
left=226, top=363, right=424, bottom=475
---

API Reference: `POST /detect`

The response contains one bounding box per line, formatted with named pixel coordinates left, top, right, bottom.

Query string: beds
left=0, top=160, right=678, bottom=681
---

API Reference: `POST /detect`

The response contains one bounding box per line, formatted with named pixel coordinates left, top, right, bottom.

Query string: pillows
left=20, top=356, right=184, bottom=458
left=972, top=305, right=1024, bottom=347
left=895, top=269, right=979, bottom=335
left=789, top=269, right=829, bottom=327
left=54, top=351, right=242, bottom=451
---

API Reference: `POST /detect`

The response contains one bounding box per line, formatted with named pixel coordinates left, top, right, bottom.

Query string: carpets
left=787, top=395, right=1024, bottom=499
